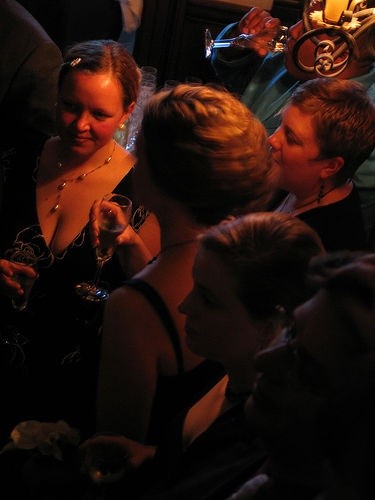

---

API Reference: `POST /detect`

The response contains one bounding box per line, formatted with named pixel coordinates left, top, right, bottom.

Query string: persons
left=226, top=249, right=375, bottom=500
left=94, top=82, right=270, bottom=447
left=0, top=0, right=375, bottom=255
left=0, top=39, right=151, bottom=499
left=79, top=212, right=328, bottom=500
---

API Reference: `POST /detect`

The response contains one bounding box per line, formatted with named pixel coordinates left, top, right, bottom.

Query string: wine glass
left=75, top=193, right=132, bottom=302
left=205, top=25, right=288, bottom=59
left=0, top=266, right=36, bottom=345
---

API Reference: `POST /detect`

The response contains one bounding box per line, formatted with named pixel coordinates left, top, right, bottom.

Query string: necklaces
left=292, top=188, right=332, bottom=209
left=53, top=136, right=115, bottom=212
left=161, top=239, right=198, bottom=253
left=225, top=381, right=251, bottom=402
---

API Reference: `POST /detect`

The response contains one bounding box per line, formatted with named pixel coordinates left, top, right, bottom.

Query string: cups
left=142, top=66, right=158, bottom=93
left=164, top=80, right=180, bottom=90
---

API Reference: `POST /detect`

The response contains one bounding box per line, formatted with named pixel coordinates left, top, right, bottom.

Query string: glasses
left=272, top=304, right=339, bottom=400
